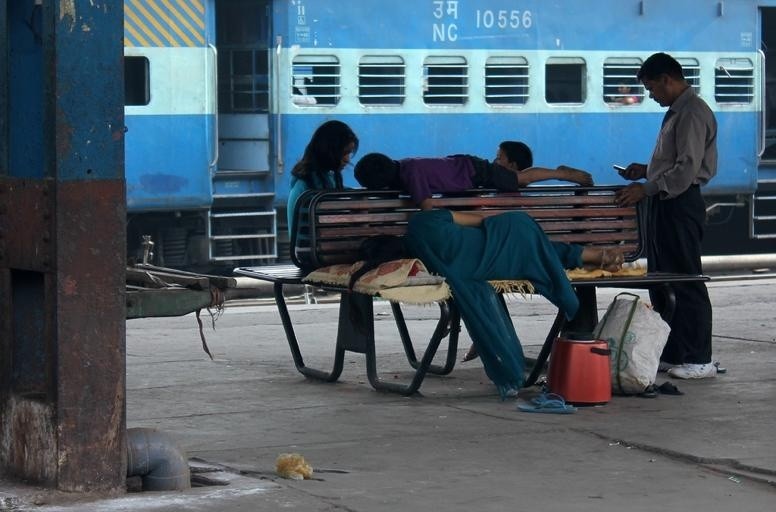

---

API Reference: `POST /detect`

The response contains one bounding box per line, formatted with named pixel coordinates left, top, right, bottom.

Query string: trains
left=124, top=0, right=776, bottom=276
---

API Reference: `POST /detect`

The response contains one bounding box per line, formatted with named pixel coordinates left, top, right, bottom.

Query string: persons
left=614, top=52, right=718, bottom=380
left=347, top=210, right=626, bottom=283
left=286, top=119, right=361, bottom=270
left=493, top=140, right=534, bottom=197
left=351, top=151, right=599, bottom=210
left=610, top=80, right=640, bottom=104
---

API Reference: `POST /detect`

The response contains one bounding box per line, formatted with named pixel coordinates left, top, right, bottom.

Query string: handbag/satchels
left=592, top=291, right=671, bottom=394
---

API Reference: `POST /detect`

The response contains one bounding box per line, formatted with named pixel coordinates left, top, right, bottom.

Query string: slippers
left=517, top=392, right=578, bottom=414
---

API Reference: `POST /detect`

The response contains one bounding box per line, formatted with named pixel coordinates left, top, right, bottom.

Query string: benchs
left=233, top=184, right=711, bottom=395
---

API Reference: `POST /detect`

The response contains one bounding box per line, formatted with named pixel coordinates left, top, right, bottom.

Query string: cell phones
left=613, top=165, right=626, bottom=172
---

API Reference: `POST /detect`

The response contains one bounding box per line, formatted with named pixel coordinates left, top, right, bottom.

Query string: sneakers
left=666, top=361, right=719, bottom=381
